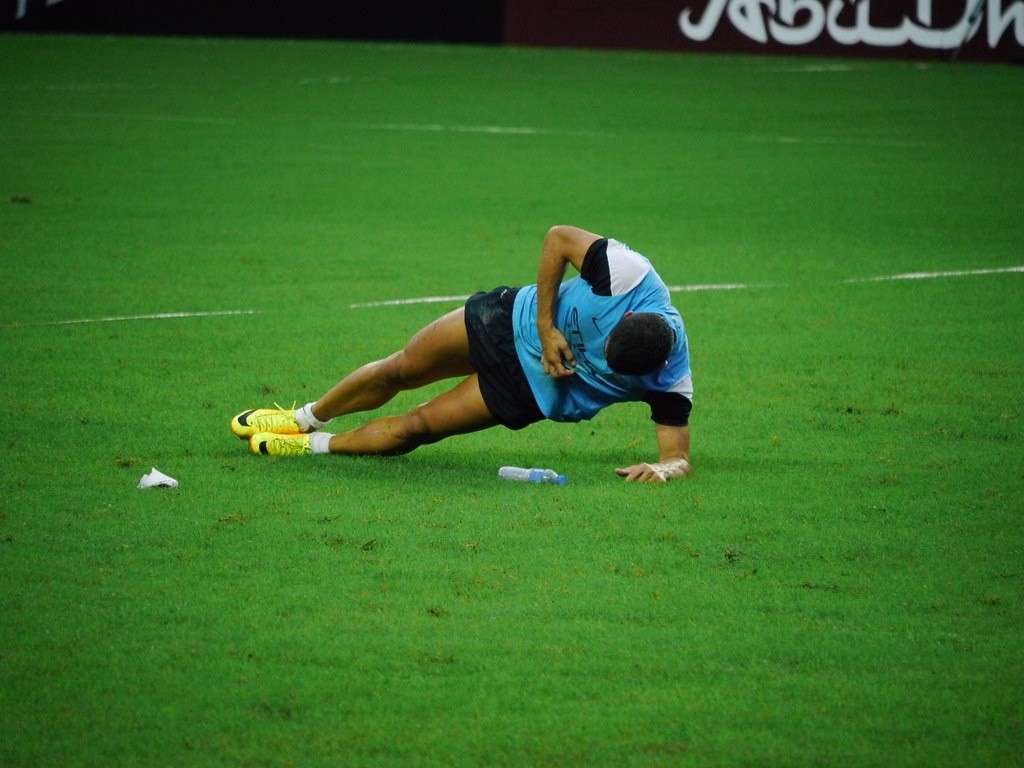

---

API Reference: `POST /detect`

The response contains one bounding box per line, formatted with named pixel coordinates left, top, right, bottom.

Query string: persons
left=231, top=226, right=693, bottom=483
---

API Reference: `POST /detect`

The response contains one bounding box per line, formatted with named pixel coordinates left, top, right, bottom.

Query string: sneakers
left=249, top=432, right=311, bottom=457
left=231, top=401, right=302, bottom=439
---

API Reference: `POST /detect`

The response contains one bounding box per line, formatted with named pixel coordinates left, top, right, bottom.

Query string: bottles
left=497, top=465, right=565, bottom=483
